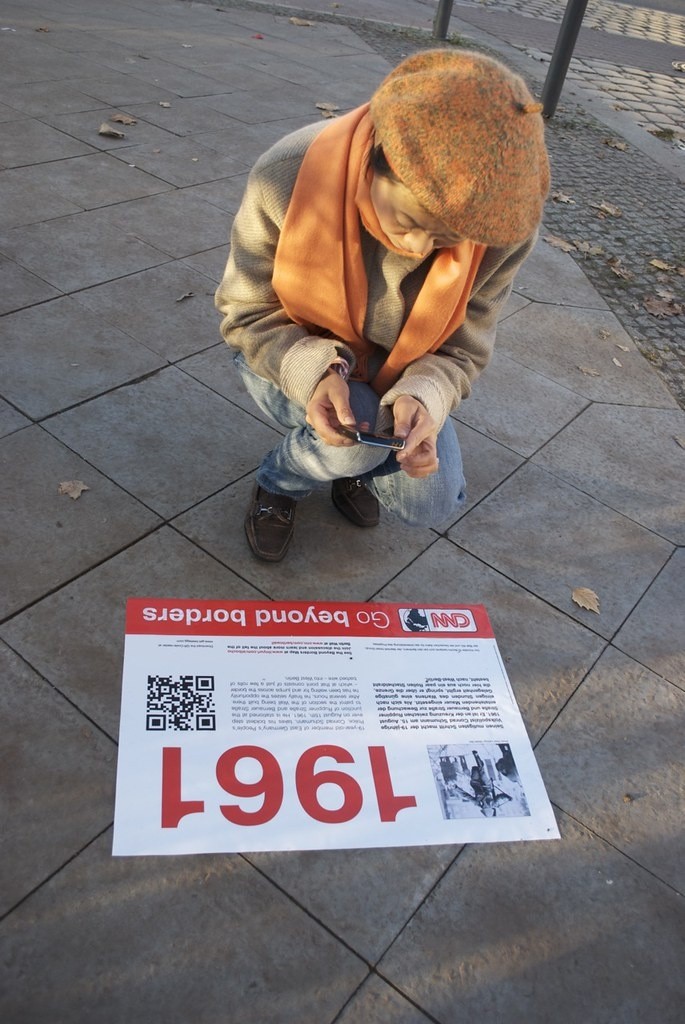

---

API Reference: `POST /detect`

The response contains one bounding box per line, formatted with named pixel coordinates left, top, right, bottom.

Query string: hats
left=370, top=47, right=548, bottom=246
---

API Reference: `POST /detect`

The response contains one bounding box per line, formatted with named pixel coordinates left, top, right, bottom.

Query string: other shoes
left=243, top=472, right=296, bottom=561
left=332, top=473, right=380, bottom=526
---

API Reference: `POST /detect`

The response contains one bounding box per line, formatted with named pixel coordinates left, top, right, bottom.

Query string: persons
left=214, top=49, right=549, bottom=563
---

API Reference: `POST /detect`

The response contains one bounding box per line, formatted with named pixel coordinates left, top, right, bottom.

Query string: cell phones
left=338, top=424, right=405, bottom=450
